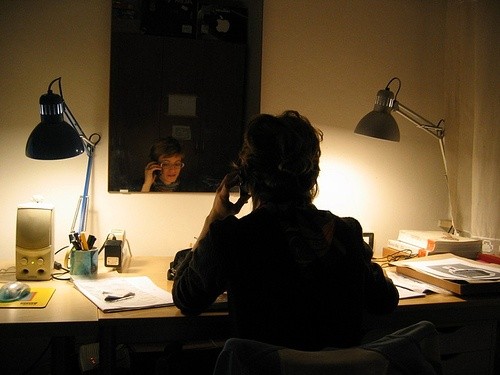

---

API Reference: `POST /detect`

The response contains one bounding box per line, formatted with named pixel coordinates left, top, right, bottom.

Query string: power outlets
left=109, top=227, right=125, bottom=248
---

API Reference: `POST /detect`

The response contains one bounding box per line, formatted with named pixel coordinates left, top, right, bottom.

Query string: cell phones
left=156, top=169, right=161, bottom=177
left=239, top=173, right=246, bottom=204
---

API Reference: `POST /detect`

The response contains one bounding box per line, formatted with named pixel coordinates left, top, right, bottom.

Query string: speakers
left=14, top=208, right=55, bottom=280
left=104, top=240, right=123, bottom=267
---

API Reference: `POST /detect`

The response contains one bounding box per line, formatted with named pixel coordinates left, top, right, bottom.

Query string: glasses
left=161, top=162, right=185, bottom=169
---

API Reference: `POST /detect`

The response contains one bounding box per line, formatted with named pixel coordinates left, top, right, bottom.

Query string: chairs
left=212, top=320, right=444, bottom=375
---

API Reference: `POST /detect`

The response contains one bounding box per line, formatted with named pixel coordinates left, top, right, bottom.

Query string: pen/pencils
left=69, top=232, right=97, bottom=250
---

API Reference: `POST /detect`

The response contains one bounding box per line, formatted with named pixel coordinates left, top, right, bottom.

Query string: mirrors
left=107, top=0, right=265, bottom=194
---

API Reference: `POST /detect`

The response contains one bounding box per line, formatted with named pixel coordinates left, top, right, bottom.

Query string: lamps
left=354, top=78, right=460, bottom=238
left=25, top=76, right=103, bottom=269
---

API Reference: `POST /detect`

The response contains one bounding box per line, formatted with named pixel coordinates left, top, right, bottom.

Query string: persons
left=171, top=111, right=399, bottom=350
left=140, top=135, right=208, bottom=194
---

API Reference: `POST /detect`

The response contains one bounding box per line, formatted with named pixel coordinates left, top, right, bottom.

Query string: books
left=73, top=275, right=175, bottom=313
left=382, top=229, right=500, bottom=299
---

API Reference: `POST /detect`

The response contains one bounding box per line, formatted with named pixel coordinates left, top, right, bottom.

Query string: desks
left=0, top=252, right=500, bottom=375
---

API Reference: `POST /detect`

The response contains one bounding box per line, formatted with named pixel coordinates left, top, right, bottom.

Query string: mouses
left=0, top=281, right=31, bottom=302
